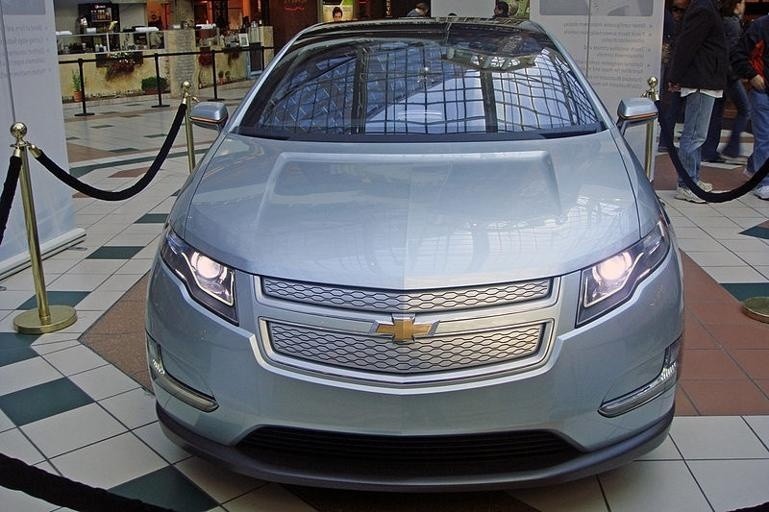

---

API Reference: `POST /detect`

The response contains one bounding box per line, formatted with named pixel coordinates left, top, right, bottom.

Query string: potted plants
left=142, top=77, right=168, bottom=94
left=71, top=70, right=82, bottom=102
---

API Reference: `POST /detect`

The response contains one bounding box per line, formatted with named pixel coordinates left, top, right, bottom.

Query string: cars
left=143, top=15, right=717, bottom=494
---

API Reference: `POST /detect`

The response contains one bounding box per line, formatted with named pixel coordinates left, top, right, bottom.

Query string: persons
left=491, top=2, right=509, bottom=18
left=406, top=3, right=429, bottom=17
left=332, top=7, right=343, bottom=22
left=656, top=0, right=769, bottom=204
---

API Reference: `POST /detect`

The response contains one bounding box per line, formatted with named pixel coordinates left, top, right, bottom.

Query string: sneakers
left=696, top=180, right=713, bottom=192
left=743, top=168, right=756, bottom=178
left=754, top=184, right=769, bottom=198
left=674, top=186, right=707, bottom=203
